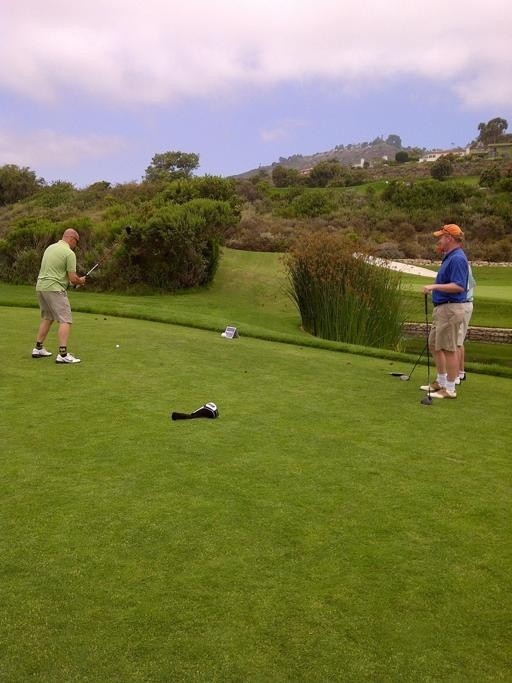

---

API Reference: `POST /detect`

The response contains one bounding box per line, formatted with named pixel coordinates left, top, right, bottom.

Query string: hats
left=433, top=224, right=462, bottom=239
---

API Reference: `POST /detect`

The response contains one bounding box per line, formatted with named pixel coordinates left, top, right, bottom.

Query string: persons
left=420, top=223, right=477, bottom=398
left=32, top=228, right=86, bottom=364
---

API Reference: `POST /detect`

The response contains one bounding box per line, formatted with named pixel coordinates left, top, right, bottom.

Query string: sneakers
left=32, top=346, right=53, bottom=358
left=55, top=352, right=80, bottom=363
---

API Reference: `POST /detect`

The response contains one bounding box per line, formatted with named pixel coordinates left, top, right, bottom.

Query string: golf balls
left=115, top=344, right=118, bottom=348
left=221, top=332, right=225, bottom=337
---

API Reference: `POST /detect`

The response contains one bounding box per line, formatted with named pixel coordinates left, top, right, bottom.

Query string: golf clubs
left=75, top=226, right=132, bottom=289
left=422, top=293, right=434, bottom=406
left=401, top=339, right=427, bottom=382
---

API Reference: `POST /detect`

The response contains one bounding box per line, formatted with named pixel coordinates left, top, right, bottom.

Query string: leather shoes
left=455, top=372, right=466, bottom=385
left=427, top=389, right=457, bottom=399
left=421, top=380, right=444, bottom=392
